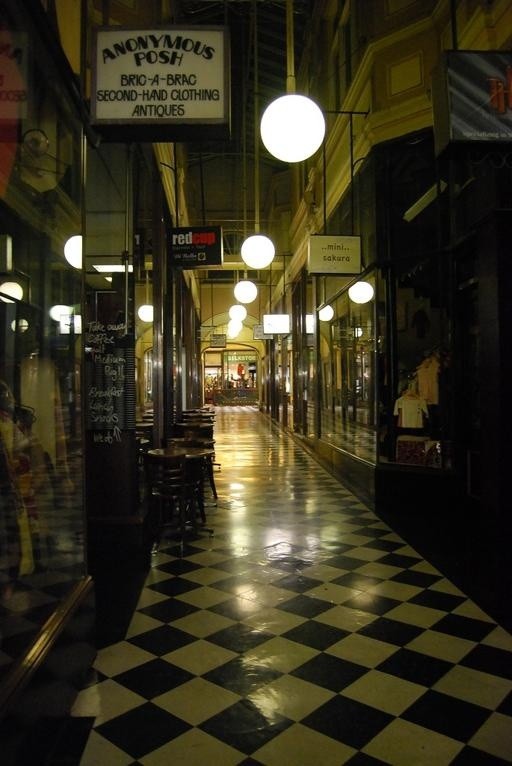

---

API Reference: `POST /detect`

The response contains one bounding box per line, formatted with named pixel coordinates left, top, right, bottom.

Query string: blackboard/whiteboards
left=83, top=317, right=124, bottom=450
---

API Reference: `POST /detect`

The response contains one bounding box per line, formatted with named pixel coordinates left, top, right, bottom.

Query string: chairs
left=135, top=408, right=153, bottom=484
left=147, top=407, right=218, bottom=546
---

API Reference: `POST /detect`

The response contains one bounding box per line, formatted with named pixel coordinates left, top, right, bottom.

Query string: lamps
left=228, top=0, right=276, bottom=339
left=317, top=276, right=334, bottom=322
left=0, top=207, right=24, bottom=305
left=347, top=280, right=374, bottom=305
left=259, top=0, right=326, bottom=164
left=137, top=271, right=154, bottom=324
